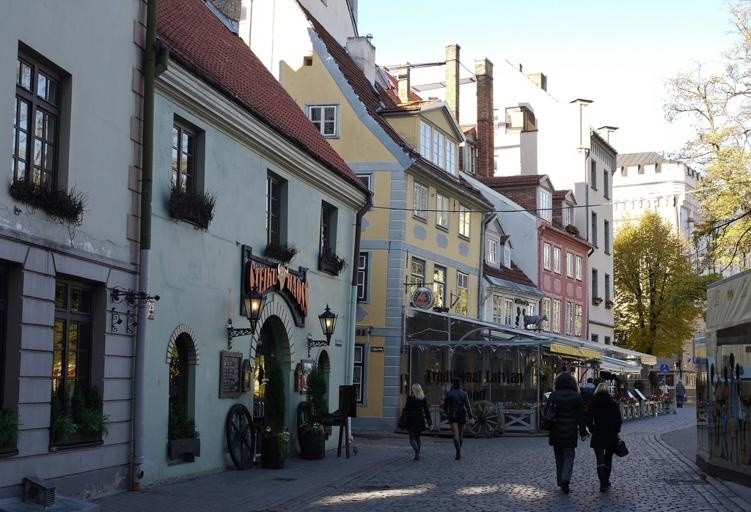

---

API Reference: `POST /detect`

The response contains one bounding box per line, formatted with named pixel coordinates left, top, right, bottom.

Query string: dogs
left=523, top=308, right=549, bottom=332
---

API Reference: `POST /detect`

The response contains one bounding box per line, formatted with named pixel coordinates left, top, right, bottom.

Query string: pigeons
left=353, top=446, right=359, bottom=455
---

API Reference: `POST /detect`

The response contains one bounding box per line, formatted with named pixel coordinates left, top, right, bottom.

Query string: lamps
left=227, top=285, right=268, bottom=348
left=308, top=304, right=337, bottom=356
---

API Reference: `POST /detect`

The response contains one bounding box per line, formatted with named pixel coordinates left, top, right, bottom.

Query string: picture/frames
left=298, top=359, right=319, bottom=395
left=218, top=350, right=244, bottom=399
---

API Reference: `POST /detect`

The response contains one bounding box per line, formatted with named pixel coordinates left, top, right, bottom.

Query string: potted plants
left=0, top=409, right=19, bottom=459
left=169, top=408, right=200, bottom=461
left=50, top=407, right=110, bottom=446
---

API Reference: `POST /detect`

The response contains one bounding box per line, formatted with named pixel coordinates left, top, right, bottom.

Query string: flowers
left=495, top=400, right=532, bottom=410
left=277, top=430, right=290, bottom=459
left=646, top=390, right=673, bottom=402
left=619, top=396, right=638, bottom=404
left=299, top=420, right=328, bottom=439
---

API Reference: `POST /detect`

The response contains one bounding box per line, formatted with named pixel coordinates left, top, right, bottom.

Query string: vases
left=261, top=437, right=287, bottom=468
left=301, top=435, right=325, bottom=460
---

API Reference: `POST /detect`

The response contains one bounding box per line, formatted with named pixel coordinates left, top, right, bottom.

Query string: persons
left=545, top=372, right=588, bottom=495
left=676, top=380, right=685, bottom=408
left=590, top=381, right=622, bottom=491
left=444, top=377, right=477, bottom=461
left=414, top=291, right=430, bottom=307
left=402, top=383, right=433, bottom=460
left=661, top=380, right=671, bottom=393
left=580, top=377, right=596, bottom=435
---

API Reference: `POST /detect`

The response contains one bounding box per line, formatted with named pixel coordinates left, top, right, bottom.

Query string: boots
left=557, top=474, right=570, bottom=494
left=409, top=439, right=421, bottom=460
left=596, top=465, right=612, bottom=492
left=453, top=438, right=464, bottom=460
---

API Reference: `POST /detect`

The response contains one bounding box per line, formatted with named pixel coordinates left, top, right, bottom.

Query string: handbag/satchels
left=615, top=438, right=629, bottom=458
left=543, top=402, right=558, bottom=431
left=398, top=412, right=407, bottom=428
